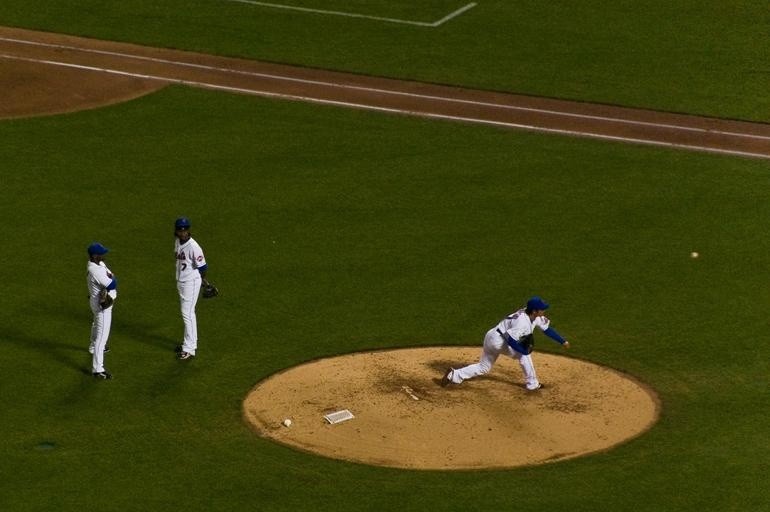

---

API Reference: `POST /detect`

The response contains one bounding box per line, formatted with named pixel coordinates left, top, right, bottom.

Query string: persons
left=441, top=296, right=571, bottom=391
left=174, top=218, right=208, bottom=360
left=86, top=243, right=117, bottom=380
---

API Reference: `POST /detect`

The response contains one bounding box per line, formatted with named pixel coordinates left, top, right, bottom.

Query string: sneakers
left=538, top=383, right=544, bottom=389
left=178, top=351, right=191, bottom=360
left=93, top=372, right=111, bottom=379
left=441, top=367, right=453, bottom=387
left=104, top=345, right=111, bottom=353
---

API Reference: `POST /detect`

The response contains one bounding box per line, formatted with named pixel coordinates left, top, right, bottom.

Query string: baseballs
left=691, top=252, right=698, bottom=257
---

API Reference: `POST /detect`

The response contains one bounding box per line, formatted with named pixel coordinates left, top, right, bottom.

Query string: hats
left=527, top=296, right=549, bottom=310
left=88, top=244, right=109, bottom=255
left=175, top=218, right=190, bottom=227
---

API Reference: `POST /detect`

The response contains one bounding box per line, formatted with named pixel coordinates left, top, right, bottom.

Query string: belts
left=497, top=329, right=502, bottom=335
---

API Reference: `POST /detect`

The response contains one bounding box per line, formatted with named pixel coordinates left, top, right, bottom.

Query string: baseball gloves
left=99, top=289, right=113, bottom=311
left=201, top=284, right=219, bottom=298
left=519, top=333, right=534, bottom=354
